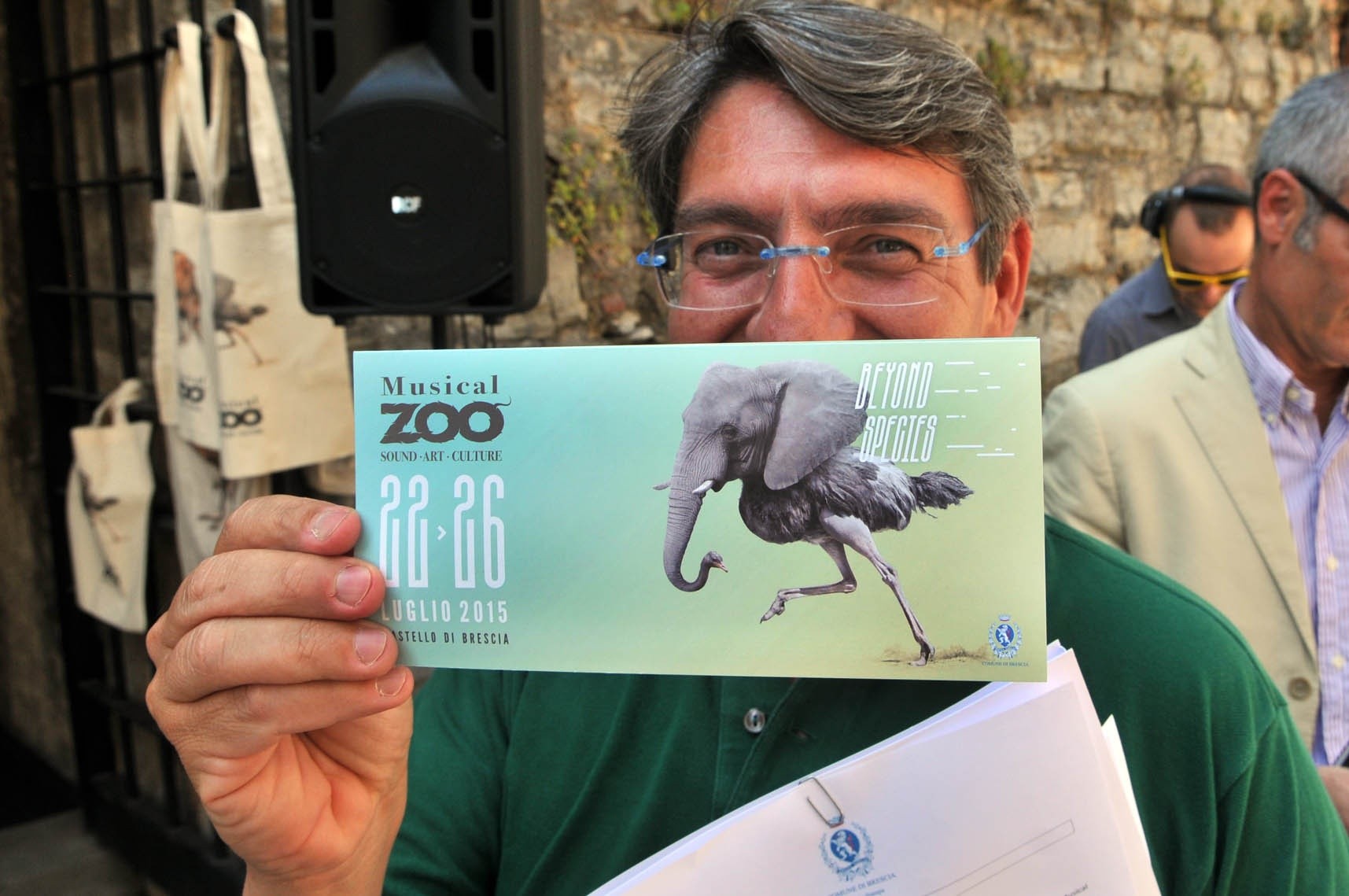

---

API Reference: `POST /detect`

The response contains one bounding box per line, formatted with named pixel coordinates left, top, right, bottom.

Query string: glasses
left=1159, top=225, right=1252, bottom=293
left=637, top=215, right=992, bottom=312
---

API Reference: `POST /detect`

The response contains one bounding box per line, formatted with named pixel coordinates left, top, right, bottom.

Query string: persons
left=1039, top=71, right=1349, bottom=844
left=1075, top=159, right=1258, bottom=375
left=143, top=0, right=1348, bottom=896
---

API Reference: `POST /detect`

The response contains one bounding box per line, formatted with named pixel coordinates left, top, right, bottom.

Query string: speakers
left=285, top=0, right=549, bottom=317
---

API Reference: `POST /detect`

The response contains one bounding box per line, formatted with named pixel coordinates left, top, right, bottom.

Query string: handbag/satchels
left=151, top=9, right=355, bottom=484
left=165, top=424, right=274, bottom=584
left=65, top=378, right=156, bottom=636
